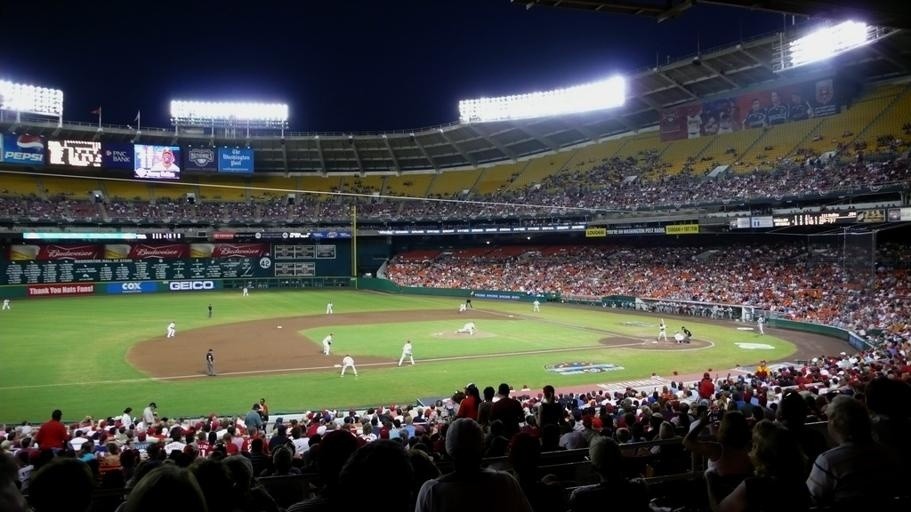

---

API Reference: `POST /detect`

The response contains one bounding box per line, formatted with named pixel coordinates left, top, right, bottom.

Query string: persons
left=676, top=89, right=813, bottom=139
left=2, top=129, right=911, bottom=512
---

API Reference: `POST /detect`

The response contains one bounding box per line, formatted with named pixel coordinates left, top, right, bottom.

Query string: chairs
left=238, top=415, right=843, bottom=511
